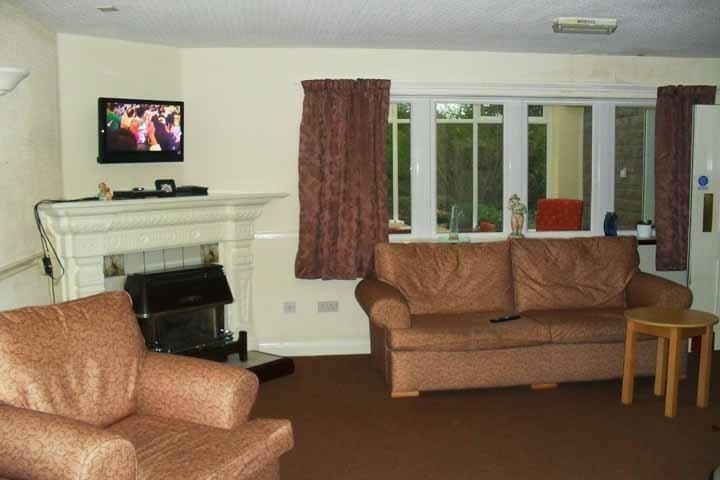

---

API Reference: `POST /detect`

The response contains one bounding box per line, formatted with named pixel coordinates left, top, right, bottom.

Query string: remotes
left=490, top=314, right=520, bottom=323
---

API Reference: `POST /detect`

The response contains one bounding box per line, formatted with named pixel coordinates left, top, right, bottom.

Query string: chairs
left=534, top=198, right=584, bottom=232
left=1, top=290, right=295, bottom=479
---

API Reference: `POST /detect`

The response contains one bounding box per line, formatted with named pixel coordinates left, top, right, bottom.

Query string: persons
left=507, top=193, right=527, bottom=239
left=106, top=103, right=181, bottom=151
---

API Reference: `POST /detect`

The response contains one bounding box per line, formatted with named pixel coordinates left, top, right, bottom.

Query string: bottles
left=603, top=212, right=618, bottom=236
left=448, top=204, right=460, bottom=239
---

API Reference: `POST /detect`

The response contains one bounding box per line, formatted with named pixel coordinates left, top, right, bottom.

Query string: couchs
left=354, top=234, right=694, bottom=400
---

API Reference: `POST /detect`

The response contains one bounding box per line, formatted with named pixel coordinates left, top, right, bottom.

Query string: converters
left=43, top=257, right=51, bottom=265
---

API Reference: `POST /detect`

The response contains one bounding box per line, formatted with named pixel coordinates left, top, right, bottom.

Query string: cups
left=636, top=224, right=652, bottom=238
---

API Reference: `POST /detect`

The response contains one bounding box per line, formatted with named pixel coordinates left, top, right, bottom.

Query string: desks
left=620, top=307, right=719, bottom=419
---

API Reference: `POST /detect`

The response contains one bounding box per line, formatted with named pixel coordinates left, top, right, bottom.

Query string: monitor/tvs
left=98, top=98, right=184, bottom=164
